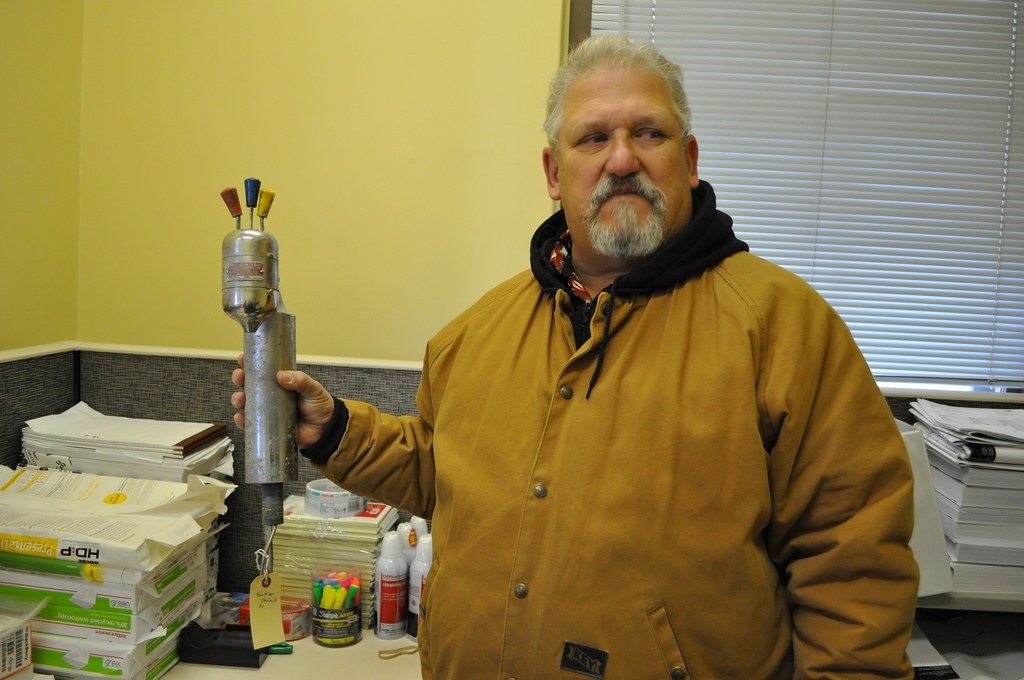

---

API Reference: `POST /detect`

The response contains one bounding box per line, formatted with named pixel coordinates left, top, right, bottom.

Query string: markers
left=312, top=572, right=363, bottom=610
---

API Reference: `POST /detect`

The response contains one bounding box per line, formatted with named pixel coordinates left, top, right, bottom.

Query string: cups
left=311, top=564, right=363, bottom=649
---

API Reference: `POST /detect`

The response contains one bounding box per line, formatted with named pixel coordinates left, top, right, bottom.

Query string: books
left=19, top=399, right=229, bottom=460
left=272, top=494, right=400, bottom=630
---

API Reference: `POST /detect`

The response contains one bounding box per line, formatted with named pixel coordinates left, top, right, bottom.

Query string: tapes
left=304, top=478, right=368, bottom=518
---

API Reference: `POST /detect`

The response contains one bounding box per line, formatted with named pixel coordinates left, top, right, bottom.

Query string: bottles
left=374, top=531, right=408, bottom=640
left=407, top=535, right=434, bottom=643
left=408, top=515, right=429, bottom=541
left=396, top=522, right=419, bottom=570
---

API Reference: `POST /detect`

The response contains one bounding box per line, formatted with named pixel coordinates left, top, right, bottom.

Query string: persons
left=231, top=32, right=920, bottom=680
